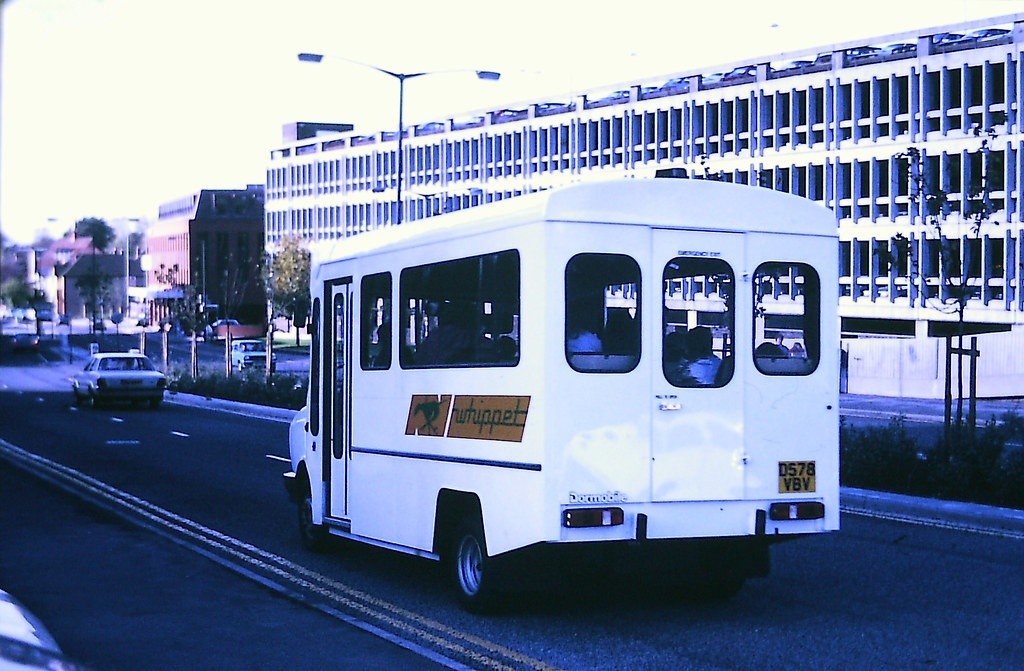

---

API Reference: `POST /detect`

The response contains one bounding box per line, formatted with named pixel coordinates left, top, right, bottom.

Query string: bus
left=280, top=177, right=842, bottom=614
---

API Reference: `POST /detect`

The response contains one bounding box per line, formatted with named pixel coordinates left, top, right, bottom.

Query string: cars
left=70, top=352, right=165, bottom=408
left=233, top=341, right=277, bottom=372
left=202, top=320, right=239, bottom=337
left=11, top=333, right=40, bottom=350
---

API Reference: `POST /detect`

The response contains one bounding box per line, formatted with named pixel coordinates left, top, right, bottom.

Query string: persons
left=370, top=295, right=805, bottom=436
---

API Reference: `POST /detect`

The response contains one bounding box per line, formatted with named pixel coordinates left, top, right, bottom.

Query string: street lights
left=48, top=215, right=141, bottom=342
left=297, top=48, right=502, bottom=228
